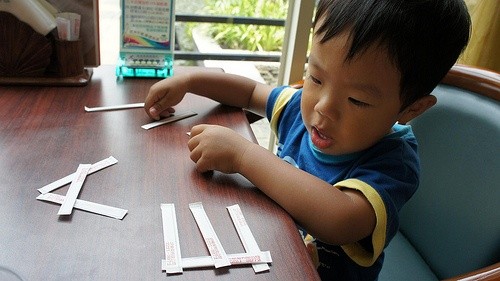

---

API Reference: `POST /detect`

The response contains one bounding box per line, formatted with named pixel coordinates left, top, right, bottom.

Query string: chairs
left=268, top=62, right=500, bottom=281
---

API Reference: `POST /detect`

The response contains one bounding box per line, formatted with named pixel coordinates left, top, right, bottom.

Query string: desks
left=0, top=64, right=321, bottom=281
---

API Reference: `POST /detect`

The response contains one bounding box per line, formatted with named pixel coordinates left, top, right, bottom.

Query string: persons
left=143, top=0, right=473, bottom=281
